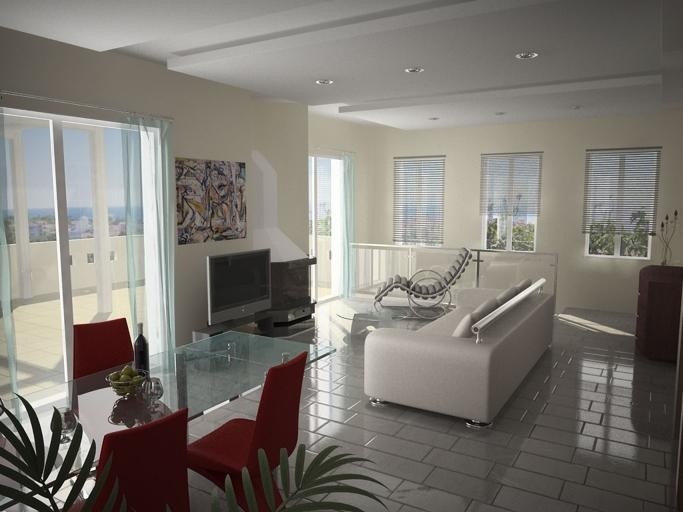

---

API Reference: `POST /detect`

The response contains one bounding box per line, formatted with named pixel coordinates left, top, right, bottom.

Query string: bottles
left=133, top=323, right=148, bottom=379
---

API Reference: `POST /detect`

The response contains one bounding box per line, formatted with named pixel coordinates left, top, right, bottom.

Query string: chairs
left=373, top=247, right=472, bottom=320
left=56, top=406, right=191, bottom=512
left=188, top=349, right=307, bottom=512
left=69, top=315, right=133, bottom=380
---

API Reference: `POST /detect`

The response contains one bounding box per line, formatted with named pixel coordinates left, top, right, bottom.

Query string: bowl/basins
left=106, top=369, right=148, bottom=395
left=107, top=397, right=148, bottom=425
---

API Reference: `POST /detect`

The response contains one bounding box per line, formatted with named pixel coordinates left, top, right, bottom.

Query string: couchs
left=364, top=277, right=555, bottom=431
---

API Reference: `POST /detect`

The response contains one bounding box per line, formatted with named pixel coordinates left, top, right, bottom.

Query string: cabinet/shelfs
left=191, top=299, right=317, bottom=372
left=634, top=264, right=683, bottom=363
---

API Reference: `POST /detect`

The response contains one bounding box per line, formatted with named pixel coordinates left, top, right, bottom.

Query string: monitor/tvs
left=207, top=248, right=272, bottom=325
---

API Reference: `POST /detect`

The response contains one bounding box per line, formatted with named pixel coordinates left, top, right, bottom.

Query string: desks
left=0, top=329, right=336, bottom=511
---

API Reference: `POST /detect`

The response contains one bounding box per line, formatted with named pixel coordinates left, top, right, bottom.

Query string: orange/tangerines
left=108, top=364, right=144, bottom=393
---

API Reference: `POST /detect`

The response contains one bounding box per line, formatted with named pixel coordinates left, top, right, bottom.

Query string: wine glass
left=140, top=377, right=163, bottom=408
left=52, top=407, right=76, bottom=443
